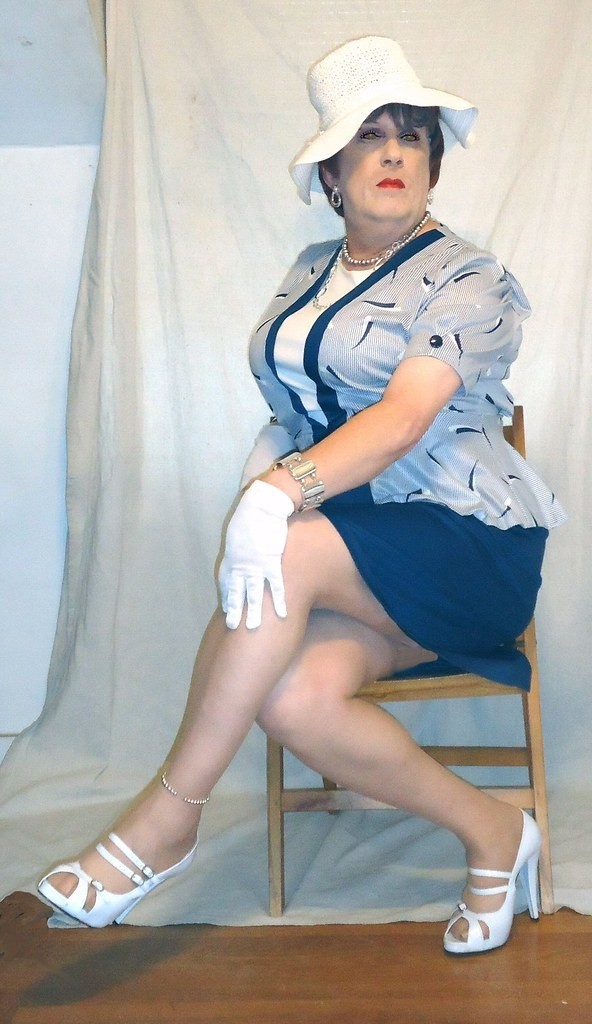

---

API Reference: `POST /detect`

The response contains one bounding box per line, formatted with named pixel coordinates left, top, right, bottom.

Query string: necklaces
left=311, top=210, right=431, bottom=312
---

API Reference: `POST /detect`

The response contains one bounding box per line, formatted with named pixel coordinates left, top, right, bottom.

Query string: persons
left=36, top=37, right=559, bottom=956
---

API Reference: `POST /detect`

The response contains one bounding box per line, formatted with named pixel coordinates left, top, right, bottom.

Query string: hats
left=288, top=35, right=479, bottom=206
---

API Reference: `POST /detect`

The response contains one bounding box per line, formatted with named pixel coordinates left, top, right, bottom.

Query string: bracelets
left=271, top=451, right=323, bottom=513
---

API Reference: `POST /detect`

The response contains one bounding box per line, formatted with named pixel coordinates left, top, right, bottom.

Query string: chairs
left=262, top=405, right=557, bottom=916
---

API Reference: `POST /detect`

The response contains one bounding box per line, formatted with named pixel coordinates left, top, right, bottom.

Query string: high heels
left=36, top=828, right=200, bottom=929
left=442, top=806, right=541, bottom=957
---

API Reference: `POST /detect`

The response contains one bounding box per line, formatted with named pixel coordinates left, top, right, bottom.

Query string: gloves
left=217, top=480, right=295, bottom=629
